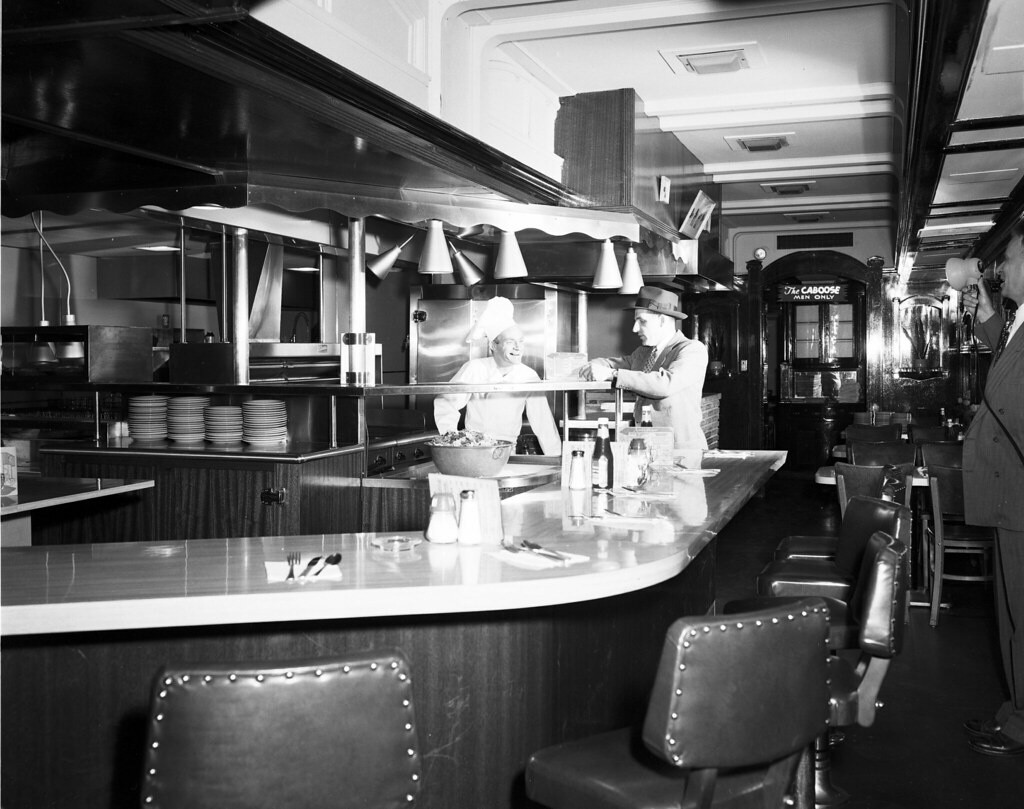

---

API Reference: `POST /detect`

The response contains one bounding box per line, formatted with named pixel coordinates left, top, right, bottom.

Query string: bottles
left=623, top=439, right=649, bottom=490
left=426, top=493, right=459, bottom=543
left=456, top=491, right=483, bottom=544
left=569, top=451, right=586, bottom=490
left=591, top=418, right=614, bottom=492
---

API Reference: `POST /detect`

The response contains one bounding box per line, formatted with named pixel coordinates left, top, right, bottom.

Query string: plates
left=242, top=400, right=288, bottom=445
left=167, top=397, right=242, bottom=443
left=854, top=411, right=911, bottom=433
left=128, top=396, right=167, bottom=441
left=372, top=537, right=421, bottom=550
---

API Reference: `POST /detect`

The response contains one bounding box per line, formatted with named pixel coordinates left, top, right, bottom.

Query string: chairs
left=834, top=423, right=994, bottom=629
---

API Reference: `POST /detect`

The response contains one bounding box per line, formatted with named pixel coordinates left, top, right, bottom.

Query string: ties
left=636, top=345, right=658, bottom=422
left=994, top=313, right=1016, bottom=366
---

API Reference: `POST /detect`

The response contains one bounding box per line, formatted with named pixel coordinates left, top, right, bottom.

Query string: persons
left=637, top=452, right=708, bottom=545
left=433, top=296, right=563, bottom=457
left=960, top=221, right=1024, bottom=755
left=578, top=286, right=709, bottom=451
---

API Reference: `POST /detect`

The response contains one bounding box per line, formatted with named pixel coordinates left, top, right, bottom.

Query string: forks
left=286, top=552, right=301, bottom=578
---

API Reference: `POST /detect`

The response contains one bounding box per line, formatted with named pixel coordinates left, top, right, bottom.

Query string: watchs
left=612, top=368, right=618, bottom=388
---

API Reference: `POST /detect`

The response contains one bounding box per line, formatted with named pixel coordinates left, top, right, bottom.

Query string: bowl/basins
left=424, top=439, right=513, bottom=475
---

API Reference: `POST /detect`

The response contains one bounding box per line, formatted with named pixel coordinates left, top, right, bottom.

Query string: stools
left=143, top=494, right=913, bottom=809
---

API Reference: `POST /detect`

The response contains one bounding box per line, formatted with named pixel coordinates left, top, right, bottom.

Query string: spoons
left=500, top=539, right=566, bottom=562
left=314, top=553, right=342, bottom=575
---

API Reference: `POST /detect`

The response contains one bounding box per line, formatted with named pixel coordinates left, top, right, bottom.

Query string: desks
left=816, top=466, right=953, bottom=609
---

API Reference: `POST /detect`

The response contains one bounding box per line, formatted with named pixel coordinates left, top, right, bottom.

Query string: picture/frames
left=678, top=189, right=716, bottom=240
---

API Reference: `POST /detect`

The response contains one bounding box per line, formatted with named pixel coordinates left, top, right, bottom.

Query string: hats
left=465, top=296, right=517, bottom=343
left=622, top=286, right=688, bottom=320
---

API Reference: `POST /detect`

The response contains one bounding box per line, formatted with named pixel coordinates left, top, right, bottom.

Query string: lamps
left=617, top=242, right=644, bottom=294
left=592, top=238, right=623, bottom=290
left=30, top=209, right=85, bottom=364
left=366, top=219, right=528, bottom=287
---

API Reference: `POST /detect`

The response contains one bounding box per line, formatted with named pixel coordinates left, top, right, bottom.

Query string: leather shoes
left=967, top=731, right=1024, bottom=758
left=963, top=714, right=1003, bottom=739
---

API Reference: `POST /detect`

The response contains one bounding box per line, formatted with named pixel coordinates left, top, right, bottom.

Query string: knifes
left=522, top=540, right=564, bottom=563
left=299, top=557, right=321, bottom=576
